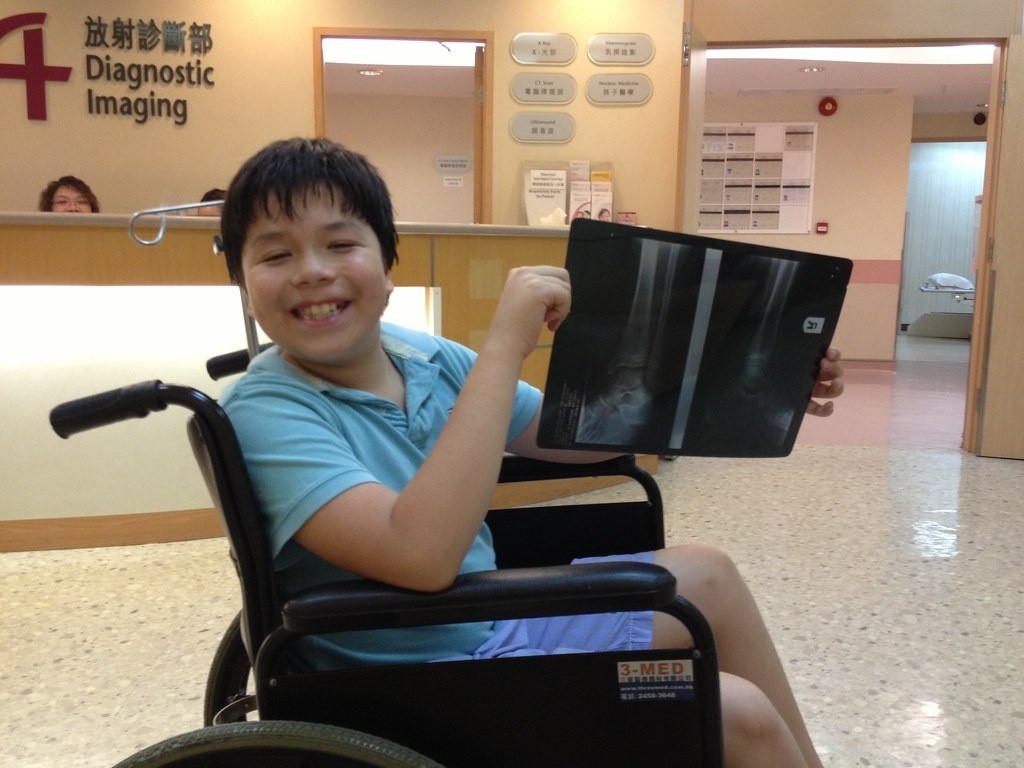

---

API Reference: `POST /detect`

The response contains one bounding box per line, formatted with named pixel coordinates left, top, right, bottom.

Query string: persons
left=577, top=211, right=591, bottom=219
left=599, top=209, right=610, bottom=222
left=198, top=188, right=226, bottom=216
left=217, top=137, right=844, bottom=768
left=39, top=176, right=99, bottom=213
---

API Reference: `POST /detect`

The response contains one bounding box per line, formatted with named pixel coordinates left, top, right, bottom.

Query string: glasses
left=52, top=197, right=92, bottom=207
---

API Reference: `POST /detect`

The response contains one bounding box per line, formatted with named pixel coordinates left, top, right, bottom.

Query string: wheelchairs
left=50, top=341, right=726, bottom=767
